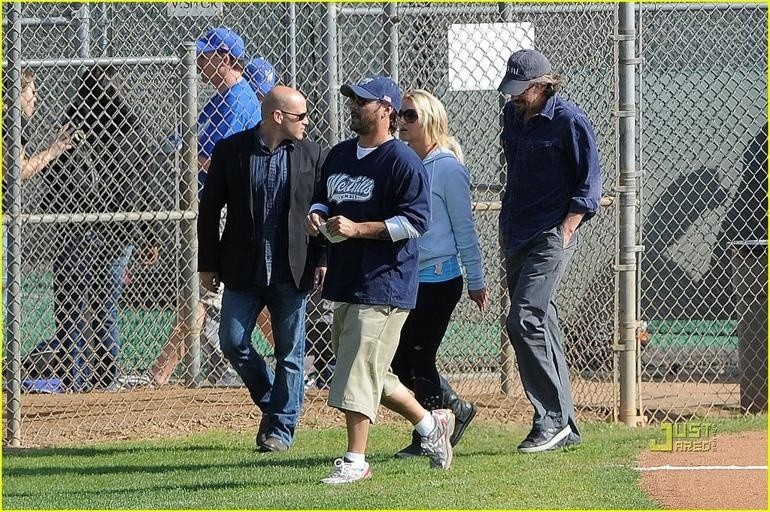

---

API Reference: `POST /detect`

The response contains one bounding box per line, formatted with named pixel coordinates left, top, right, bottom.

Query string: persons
left=302, top=77, right=456, bottom=485
left=197, top=85, right=328, bottom=452
left=391, top=90, right=490, bottom=459
left=498, top=51, right=601, bottom=453
left=2, top=29, right=276, bottom=388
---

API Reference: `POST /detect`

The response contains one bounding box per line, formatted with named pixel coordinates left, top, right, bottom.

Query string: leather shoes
left=256, top=417, right=287, bottom=451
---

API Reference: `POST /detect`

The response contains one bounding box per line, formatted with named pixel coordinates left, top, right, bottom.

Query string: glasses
left=272, top=110, right=306, bottom=120
left=399, top=110, right=418, bottom=123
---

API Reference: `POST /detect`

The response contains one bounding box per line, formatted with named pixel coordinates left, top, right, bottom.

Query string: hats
left=497, top=49, right=551, bottom=96
left=340, top=76, right=401, bottom=114
left=196, top=28, right=274, bottom=94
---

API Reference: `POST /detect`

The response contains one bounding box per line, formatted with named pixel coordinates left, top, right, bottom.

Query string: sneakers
left=319, top=456, right=372, bottom=483
left=420, top=409, right=455, bottom=470
left=116, top=375, right=159, bottom=390
left=517, top=423, right=571, bottom=453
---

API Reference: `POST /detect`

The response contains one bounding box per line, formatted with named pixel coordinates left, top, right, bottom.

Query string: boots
left=394, top=374, right=476, bottom=458
